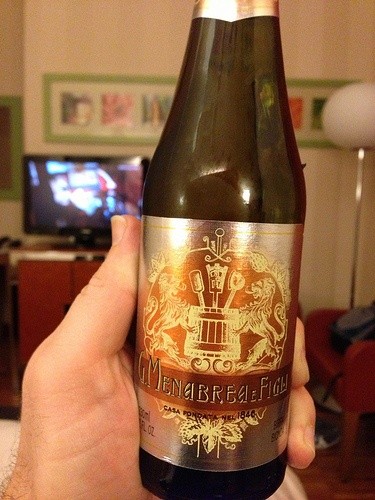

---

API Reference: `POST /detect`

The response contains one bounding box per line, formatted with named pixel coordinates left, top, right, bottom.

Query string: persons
left=0, top=214, right=316, bottom=500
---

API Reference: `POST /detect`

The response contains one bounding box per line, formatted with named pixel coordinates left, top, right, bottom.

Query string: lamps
left=322, top=81, right=375, bottom=308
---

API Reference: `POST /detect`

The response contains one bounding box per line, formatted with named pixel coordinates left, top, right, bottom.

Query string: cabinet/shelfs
left=2, top=245, right=117, bottom=424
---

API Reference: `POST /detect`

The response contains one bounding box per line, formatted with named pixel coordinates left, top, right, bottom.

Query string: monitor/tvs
left=22, top=155, right=150, bottom=251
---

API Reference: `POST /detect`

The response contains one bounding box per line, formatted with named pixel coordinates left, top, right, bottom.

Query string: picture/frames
left=0, top=95, right=23, bottom=201
left=43, top=72, right=360, bottom=147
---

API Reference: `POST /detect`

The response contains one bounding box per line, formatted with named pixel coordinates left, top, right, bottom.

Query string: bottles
left=133, top=0, right=307, bottom=500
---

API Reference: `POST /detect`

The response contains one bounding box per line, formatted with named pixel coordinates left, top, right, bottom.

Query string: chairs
left=306, top=308, right=375, bottom=480
